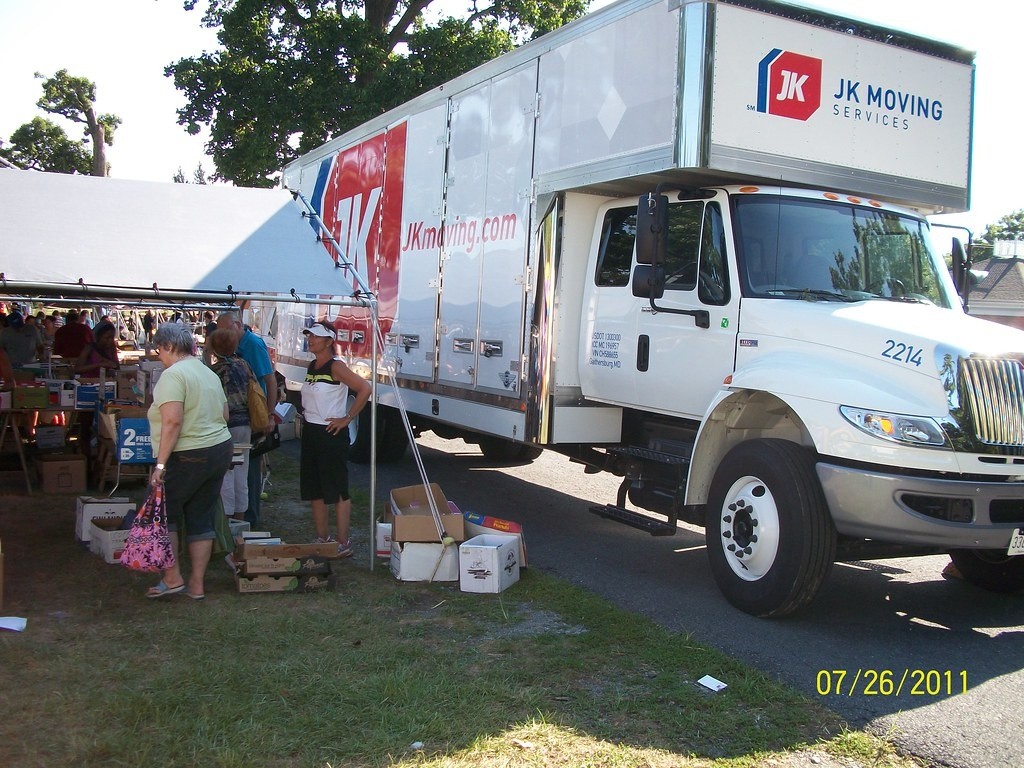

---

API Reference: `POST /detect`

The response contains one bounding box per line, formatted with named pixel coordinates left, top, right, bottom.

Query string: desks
left=51, top=351, right=161, bottom=364
left=0, top=408, right=153, bottom=496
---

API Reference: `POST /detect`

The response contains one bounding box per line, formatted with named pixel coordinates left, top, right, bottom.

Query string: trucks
left=275, top=1, right=1023, bottom=619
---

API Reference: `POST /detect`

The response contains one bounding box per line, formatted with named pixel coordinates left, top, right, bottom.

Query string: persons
left=11, top=302, right=26, bottom=320
left=33, top=302, right=46, bottom=317
left=76, top=320, right=120, bottom=378
left=0, top=312, right=47, bottom=363
left=39, top=316, right=57, bottom=363
left=0, top=305, right=111, bottom=332
left=202, top=311, right=218, bottom=366
left=301, top=321, right=372, bottom=560
left=129, top=309, right=168, bottom=342
left=143, top=322, right=235, bottom=599
left=54, top=310, right=93, bottom=358
left=207, top=328, right=258, bottom=520
left=217, top=312, right=277, bottom=528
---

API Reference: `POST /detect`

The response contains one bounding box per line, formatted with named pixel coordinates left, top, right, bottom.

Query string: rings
left=153, top=479, right=157, bottom=482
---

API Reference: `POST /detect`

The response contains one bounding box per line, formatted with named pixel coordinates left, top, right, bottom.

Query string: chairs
left=789, top=255, right=844, bottom=288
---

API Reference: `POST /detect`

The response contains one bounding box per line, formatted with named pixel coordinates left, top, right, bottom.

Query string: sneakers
left=336, top=536, right=353, bottom=559
left=310, top=535, right=332, bottom=544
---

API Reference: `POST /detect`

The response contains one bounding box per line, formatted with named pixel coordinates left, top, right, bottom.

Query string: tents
left=0, top=167, right=379, bottom=570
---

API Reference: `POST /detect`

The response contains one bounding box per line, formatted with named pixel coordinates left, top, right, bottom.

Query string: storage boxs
left=374, top=483, right=529, bottom=594
left=27, top=442, right=87, bottom=495
left=0, top=355, right=165, bottom=410
left=99, top=408, right=159, bottom=464
left=279, top=422, right=295, bottom=441
left=72, top=494, right=138, bottom=565
left=34, top=426, right=68, bottom=448
left=227, top=517, right=340, bottom=592
left=116, top=340, right=134, bottom=351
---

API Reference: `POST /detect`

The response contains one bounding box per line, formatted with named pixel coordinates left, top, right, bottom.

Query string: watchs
left=156, top=463, right=166, bottom=470
left=346, top=414, right=354, bottom=420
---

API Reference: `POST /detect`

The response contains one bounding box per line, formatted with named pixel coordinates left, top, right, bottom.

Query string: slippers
left=178, top=585, right=205, bottom=600
left=144, top=578, right=186, bottom=597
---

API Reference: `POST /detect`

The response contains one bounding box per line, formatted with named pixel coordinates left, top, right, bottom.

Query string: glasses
left=155, top=346, right=161, bottom=355
left=306, top=333, right=330, bottom=338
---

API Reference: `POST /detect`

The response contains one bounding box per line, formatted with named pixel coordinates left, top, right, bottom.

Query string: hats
left=9, top=313, right=24, bottom=329
left=302, top=322, right=336, bottom=341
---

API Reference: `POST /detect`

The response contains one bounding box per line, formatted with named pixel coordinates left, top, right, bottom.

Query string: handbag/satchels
left=239, top=357, right=269, bottom=431
left=119, top=484, right=176, bottom=573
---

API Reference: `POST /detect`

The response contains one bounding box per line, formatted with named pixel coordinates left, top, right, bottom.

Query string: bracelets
left=268, top=412, right=275, bottom=416
